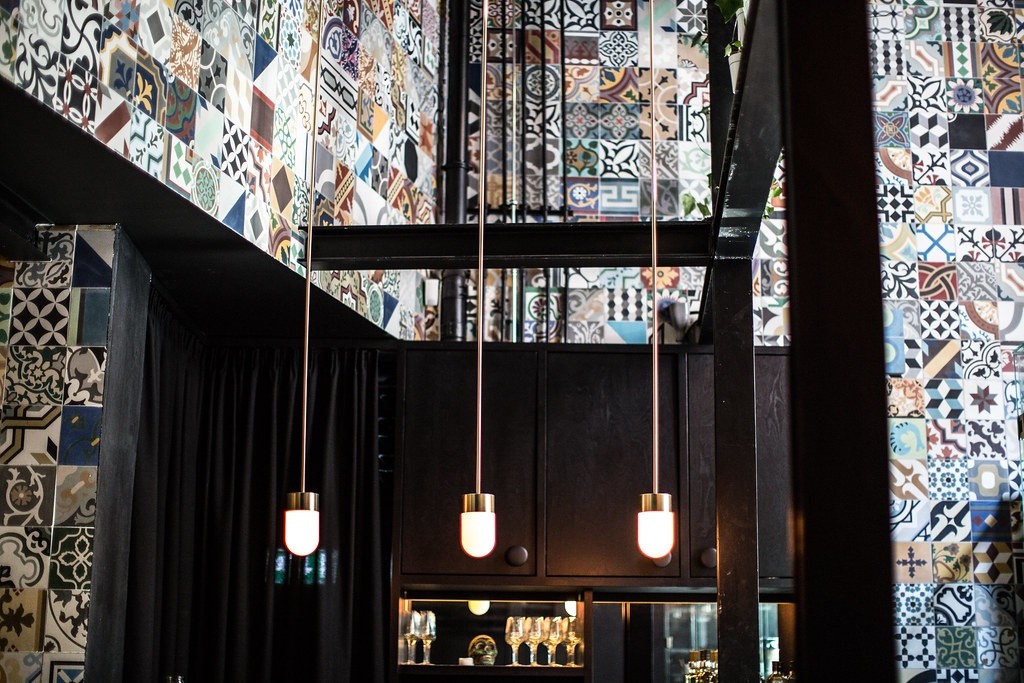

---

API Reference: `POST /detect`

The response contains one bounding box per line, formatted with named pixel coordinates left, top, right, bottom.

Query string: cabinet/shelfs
left=392, top=340, right=547, bottom=603
left=544, top=343, right=793, bottom=603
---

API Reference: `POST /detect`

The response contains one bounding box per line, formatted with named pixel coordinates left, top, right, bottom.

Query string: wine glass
left=544, top=616, right=564, bottom=666
left=525, top=617, right=544, bottom=667
left=416, top=610, right=438, bottom=666
left=505, top=616, right=526, bottom=666
left=562, top=616, right=583, bottom=667
left=400, top=611, right=419, bottom=664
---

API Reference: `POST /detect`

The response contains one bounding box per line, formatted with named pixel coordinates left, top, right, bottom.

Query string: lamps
left=636, top=0, right=677, bottom=559
left=460, top=0, right=497, bottom=558
left=283, top=0, right=322, bottom=557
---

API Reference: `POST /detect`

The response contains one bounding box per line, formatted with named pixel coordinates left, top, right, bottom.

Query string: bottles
left=777, top=662, right=785, bottom=681
left=684, top=649, right=719, bottom=683
left=767, top=662, right=785, bottom=683
left=786, top=660, right=797, bottom=683
left=168, top=675, right=183, bottom=683
